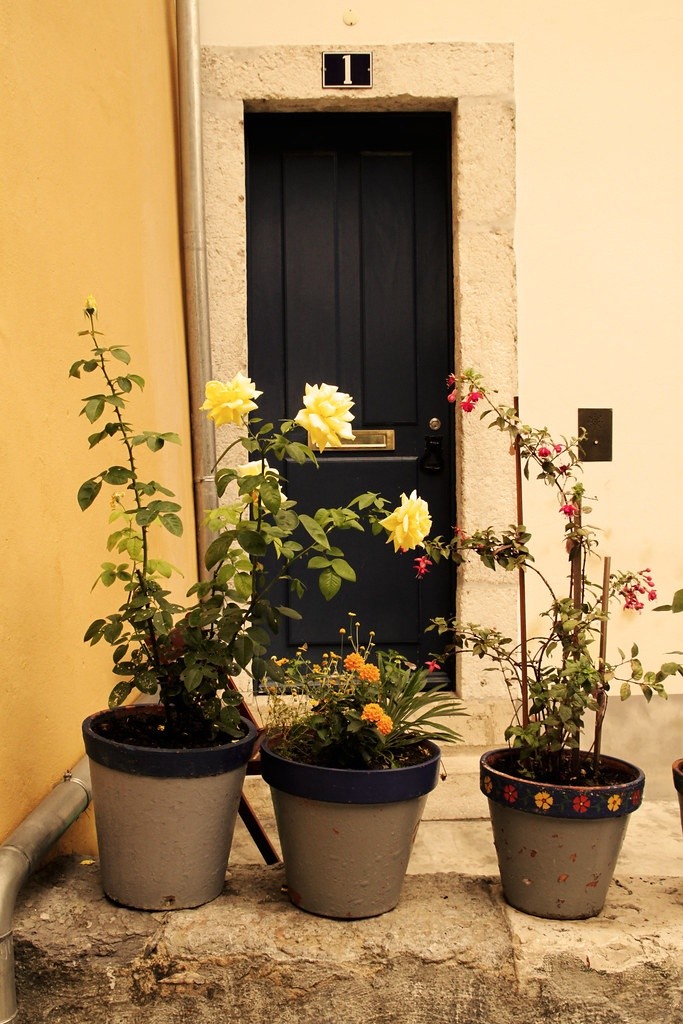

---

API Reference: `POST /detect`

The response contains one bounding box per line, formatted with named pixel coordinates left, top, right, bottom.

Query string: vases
left=80, top=706, right=251, bottom=907
left=475, top=738, right=645, bottom=915
left=259, top=726, right=439, bottom=921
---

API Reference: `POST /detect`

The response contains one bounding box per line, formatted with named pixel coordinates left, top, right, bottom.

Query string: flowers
left=67, top=294, right=432, bottom=716
left=412, top=371, right=678, bottom=751
left=263, top=614, right=468, bottom=763
